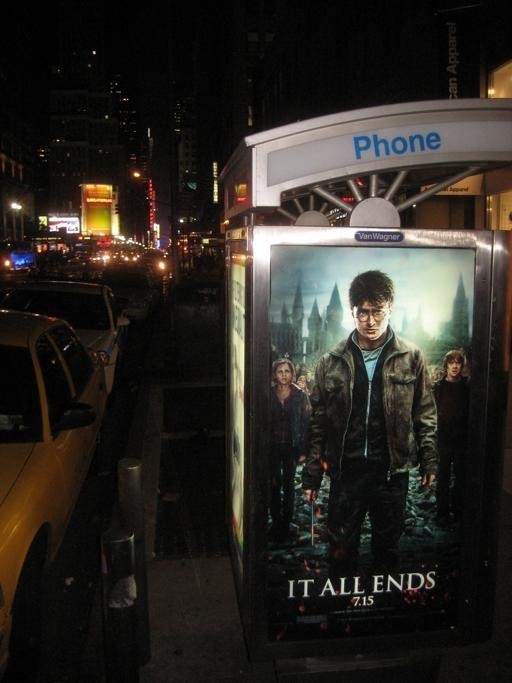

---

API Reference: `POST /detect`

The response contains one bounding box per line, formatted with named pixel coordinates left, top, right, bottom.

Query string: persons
left=300, top=267, right=440, bottom=613
left=431, top=347, right=472, bottom=529
left=267, top=354, right=312, bottom=544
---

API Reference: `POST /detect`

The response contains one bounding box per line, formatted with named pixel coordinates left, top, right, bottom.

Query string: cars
left=0, top=237, right=225, bottom=278
left=0, top=271, right=132, bottom=400
left=0, top=306, right=114, bottom=683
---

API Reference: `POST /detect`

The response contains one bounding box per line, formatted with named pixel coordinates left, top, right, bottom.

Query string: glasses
left=352, top=309, right=392, bottom=322
